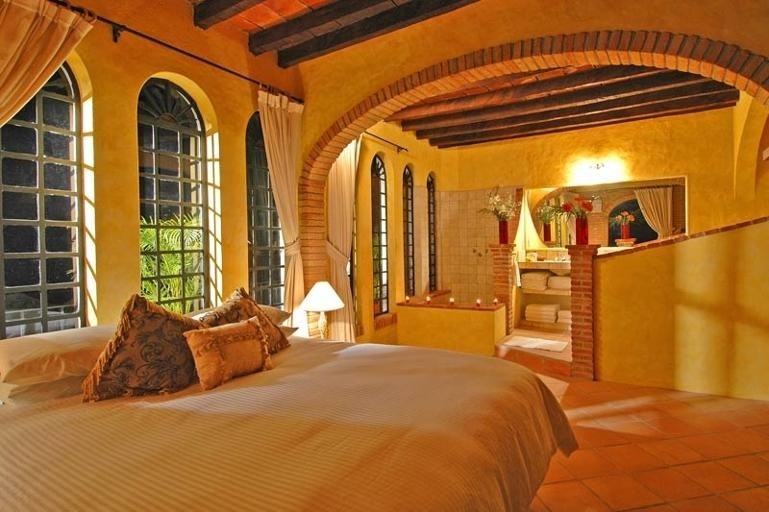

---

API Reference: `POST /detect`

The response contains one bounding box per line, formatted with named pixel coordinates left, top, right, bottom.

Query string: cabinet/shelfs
left=514, top=267, right=571, bottom=334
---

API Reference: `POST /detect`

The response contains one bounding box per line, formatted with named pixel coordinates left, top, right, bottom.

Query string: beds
left=1, top=337, right=579, bottom=512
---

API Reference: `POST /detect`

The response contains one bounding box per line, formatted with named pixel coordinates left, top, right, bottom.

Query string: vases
left=560, top=221, right=568, bottom=241
left=543, top=223, right=551, bottom=242
left=621, top=223, right=631, bottom=239
left=498, top=218, right=508, bottom=246
left=576, top=216, right=588, bottom=245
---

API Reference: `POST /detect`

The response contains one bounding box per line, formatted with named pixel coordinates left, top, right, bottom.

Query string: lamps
left=300, top=279, right=346, bottom=340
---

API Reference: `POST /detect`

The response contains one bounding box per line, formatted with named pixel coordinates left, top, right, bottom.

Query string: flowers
left=535, top=204, right=554, bottom=222
left=552, top=208, right=570, bottom=222
left=561, top=194, right=599, bottom=216
left=615, top=210, right=635, bottom=224
left=476, top=183, right=521, bottom=219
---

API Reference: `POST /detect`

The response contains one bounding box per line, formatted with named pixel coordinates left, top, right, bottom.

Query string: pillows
left=183, top=315, right=274, bottom=391
left=0, top=326, right=116, bottom=385
left=280, top=323, right=300, bottom=337
left=80, top=295, right=211, bottom=401
left=260, top=303, right=292, bottom=323
left=197, top=289, right=289, bottom=354
